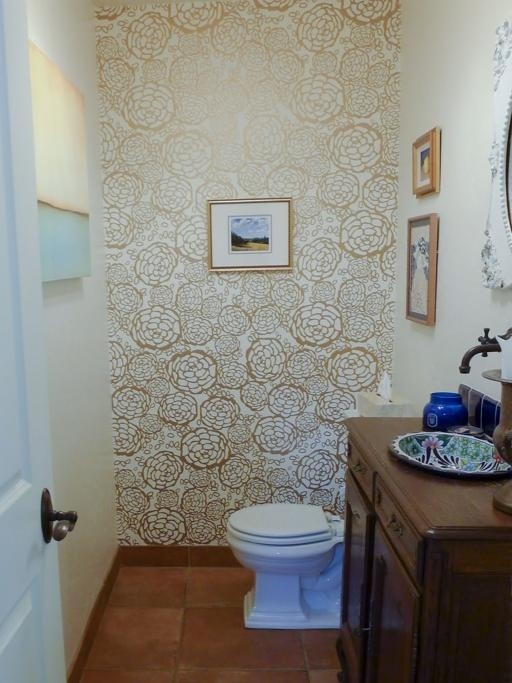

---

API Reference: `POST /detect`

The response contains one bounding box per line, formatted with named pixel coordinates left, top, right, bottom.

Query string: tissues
left=356, top=374, right=413, bottom=418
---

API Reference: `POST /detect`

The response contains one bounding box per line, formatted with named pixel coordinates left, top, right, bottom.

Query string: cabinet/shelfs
left=335, top=434, right=512, bottom=679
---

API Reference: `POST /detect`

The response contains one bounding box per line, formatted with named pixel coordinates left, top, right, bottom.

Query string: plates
left=388, top=430, right=512, bottom=480
left=447, top=425, right=484, bottom=436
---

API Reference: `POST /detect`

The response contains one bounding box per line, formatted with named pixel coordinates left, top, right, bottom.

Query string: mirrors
left=479, top=16, right=512, bottom=288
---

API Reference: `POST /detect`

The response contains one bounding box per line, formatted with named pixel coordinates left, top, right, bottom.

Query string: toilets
left=227, top=408, right=359, bottom=630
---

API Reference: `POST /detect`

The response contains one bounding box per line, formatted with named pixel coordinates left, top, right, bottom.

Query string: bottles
left=422, top=390, right=469, bottom=430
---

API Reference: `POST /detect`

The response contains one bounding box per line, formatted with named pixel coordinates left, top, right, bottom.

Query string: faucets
left=458, top=328, right=501, bottom=377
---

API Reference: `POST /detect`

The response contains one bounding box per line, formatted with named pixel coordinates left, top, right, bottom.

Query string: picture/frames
left=207, top=198, right=293, bottom=272
left=410, top=128, right=442, bottom=197
left=405, top=213, right=438, bottom=326
